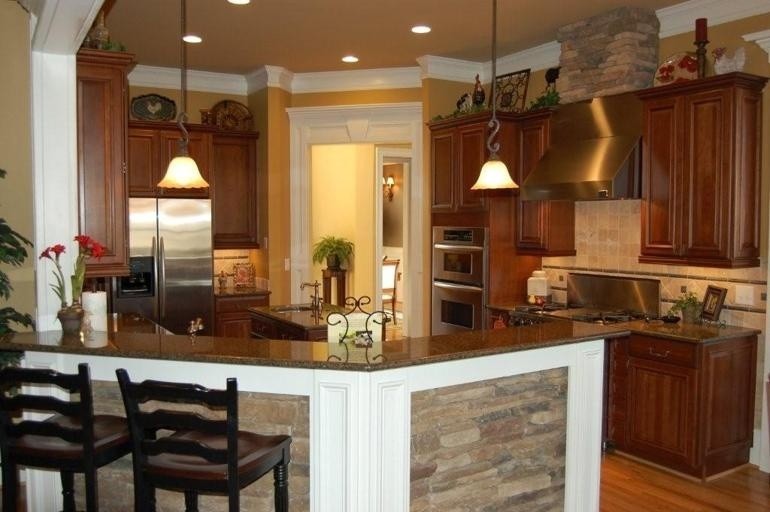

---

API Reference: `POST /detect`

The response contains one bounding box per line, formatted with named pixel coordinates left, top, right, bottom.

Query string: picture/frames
left=233, top=263, right=255, bottom=288
left=700, top=284, right=727, bottom=321
left=117, top=257, right=154, bottom=296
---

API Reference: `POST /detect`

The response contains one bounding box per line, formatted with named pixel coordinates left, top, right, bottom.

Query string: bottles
left=93, top=10, right=109, bottom=50
left=217, top=271, right=228, bottom=289
left=527, top=270, right=551, bottom=310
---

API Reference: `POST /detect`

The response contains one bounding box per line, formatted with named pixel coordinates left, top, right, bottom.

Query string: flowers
left=39, top=235, right=105, bottom=315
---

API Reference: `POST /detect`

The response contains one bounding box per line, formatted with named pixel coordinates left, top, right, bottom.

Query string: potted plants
left=312, top=236, right=354, bottom=270
left=668, top=291, right=703, bottom=324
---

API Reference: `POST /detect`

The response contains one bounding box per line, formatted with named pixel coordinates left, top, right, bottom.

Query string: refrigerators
left=112, top=196, right=215, bottom=336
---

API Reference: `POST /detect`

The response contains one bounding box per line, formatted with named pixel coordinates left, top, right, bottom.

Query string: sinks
left=271, top=305, right=312, bottom=314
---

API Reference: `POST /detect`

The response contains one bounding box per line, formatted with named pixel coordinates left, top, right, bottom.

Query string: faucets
left=300, top=280, right=322, bottom=308
left=300, top=309, right=323, bottom=325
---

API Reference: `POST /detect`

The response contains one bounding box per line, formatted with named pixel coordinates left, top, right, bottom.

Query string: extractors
left=520, top=92, right=646, bottom=201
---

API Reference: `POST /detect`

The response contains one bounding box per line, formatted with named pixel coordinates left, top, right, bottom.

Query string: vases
left=56, top=314, right=84, bottom=335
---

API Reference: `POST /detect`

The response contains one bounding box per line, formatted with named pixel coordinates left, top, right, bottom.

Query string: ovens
left=431, top=226, right=484, bottom=337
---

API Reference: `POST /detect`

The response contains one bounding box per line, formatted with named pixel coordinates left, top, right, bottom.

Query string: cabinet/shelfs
left=607, top=328, right=760, bottom=483
left=428, top=110, right=543, bottom=217
left=129, top=119, right=209, bottom=199
left=513, top=109, right=577, bottom=254
left=637, top=72, right=770, bottom=269
left=214, top=285, right=271, bottom=340
left=76, top=48, right=138, bottom=277
left=210, top=126, right=260, bottom=249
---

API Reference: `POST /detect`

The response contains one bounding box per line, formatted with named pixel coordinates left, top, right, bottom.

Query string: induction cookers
left=572, top=309, right=645, bottom=326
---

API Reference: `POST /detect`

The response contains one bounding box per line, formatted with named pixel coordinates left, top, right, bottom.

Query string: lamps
left=382, top=175, right=394, bottom=203
left=470, top=0, right=519, bottom=191
left=157, top=0, right=212, bottom=190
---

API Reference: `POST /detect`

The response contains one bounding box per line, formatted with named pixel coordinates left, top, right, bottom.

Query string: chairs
left=115, top=368, right=292, bottom=512
left=325, top=312, right=382, bottom=344
left=0, top=363, right=134, bottom=512
left=382, top=259, right=400, bottom=325
